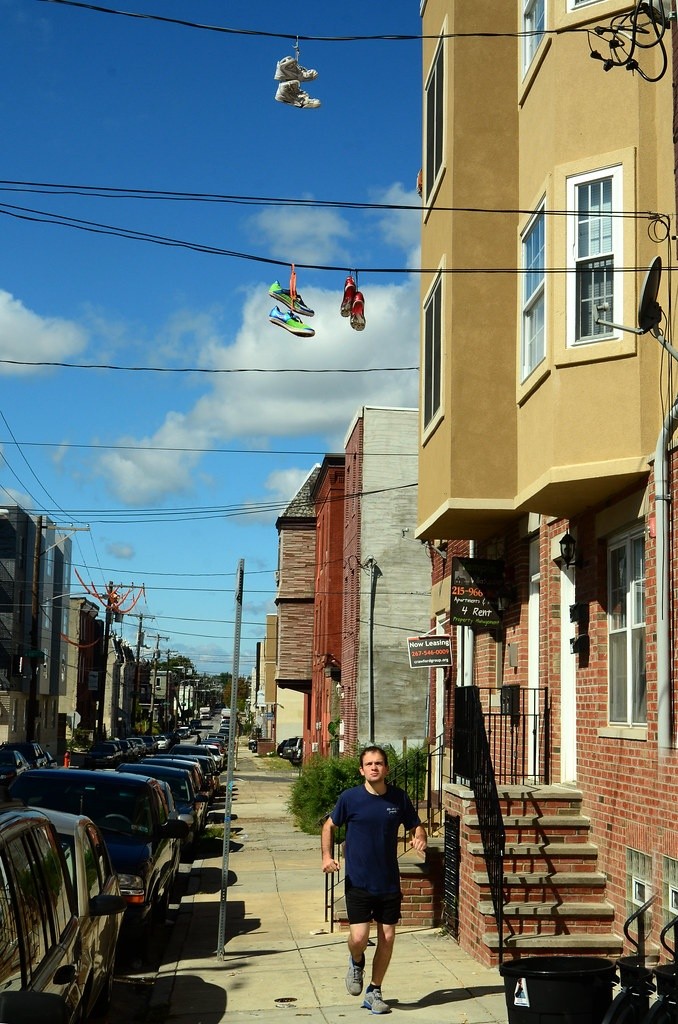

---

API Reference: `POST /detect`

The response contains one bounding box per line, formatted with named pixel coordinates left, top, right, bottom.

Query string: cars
left=1, top=809, right=85, bottom=1024
left=276, top=735, right=304, bottom=766
left=0, top=705, right=239, bottom=851
left=1, top=765, right=190, bottom=966
left=1, top=804, right=126, bottom=1024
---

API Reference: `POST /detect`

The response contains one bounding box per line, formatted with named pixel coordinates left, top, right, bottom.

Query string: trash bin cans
left=498, top=955, right=615, bottom=1024
left=256, top=738, right=276, bottom=756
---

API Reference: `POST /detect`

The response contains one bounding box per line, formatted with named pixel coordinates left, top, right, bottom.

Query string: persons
left=196, top=735, right=202, bottom=745
left=320, top=746, right=428, bottom=1013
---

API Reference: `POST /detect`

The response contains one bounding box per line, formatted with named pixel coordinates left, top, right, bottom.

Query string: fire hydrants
left=63, top=752, right=70, bottom=769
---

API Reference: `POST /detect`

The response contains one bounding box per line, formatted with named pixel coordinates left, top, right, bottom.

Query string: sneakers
left=274, top=56, right=318, bottom=82
left=269, top=280, right=314, bottom=316
left=364, top=985, right=390, bottom=1014
left=345, top=954, right=366, bottom=996
left=275, top=80, right=321, bottom=109
left=350, top=292, right=366, bottom=331
left=340, top=276, right=356, bottom=317
left=268, top=305, right=315, bottom=337
